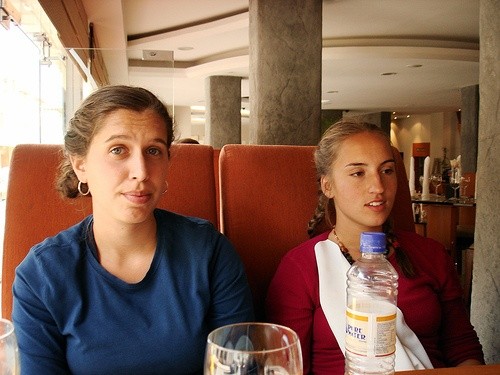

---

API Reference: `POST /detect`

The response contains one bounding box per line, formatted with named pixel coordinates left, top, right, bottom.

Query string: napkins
left=422, top=156, right=431, bottom=200
left=314, top=239, right=434, bottom=372
left=409, top=156, right=415, bottom=196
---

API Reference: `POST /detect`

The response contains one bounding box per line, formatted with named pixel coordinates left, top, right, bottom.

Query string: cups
left=419, top=176, right=431, bottom=187
left=204, top=322, right=303, bottom=375
left=0, top=319, right=21, bottom=375
left=412, top=190, right=420, bottom=200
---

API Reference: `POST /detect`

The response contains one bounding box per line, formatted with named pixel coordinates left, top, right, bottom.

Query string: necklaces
left=332, top=228, right=389, bottom=265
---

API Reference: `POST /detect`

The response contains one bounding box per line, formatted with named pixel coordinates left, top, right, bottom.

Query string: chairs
left=1, top=140, right=416, bottom=333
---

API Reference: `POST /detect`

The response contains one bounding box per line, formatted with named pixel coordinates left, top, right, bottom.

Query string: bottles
left=344, top=232, right=399, bottom=375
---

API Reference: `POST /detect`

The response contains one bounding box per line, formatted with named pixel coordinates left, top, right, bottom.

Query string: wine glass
left=431, top=176, right=442, bottom=197
left=449, top=178, right=460, bottom=200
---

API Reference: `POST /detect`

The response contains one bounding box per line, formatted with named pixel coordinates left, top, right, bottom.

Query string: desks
left=411, top=192, right=476, bottom=253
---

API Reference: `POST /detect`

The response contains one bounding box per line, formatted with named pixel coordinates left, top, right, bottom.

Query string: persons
left=12, top=85, right=253, bottom=375
left=178, top=138, right=199, bottom=144
left=267, top=121, right=483, bottom=375
